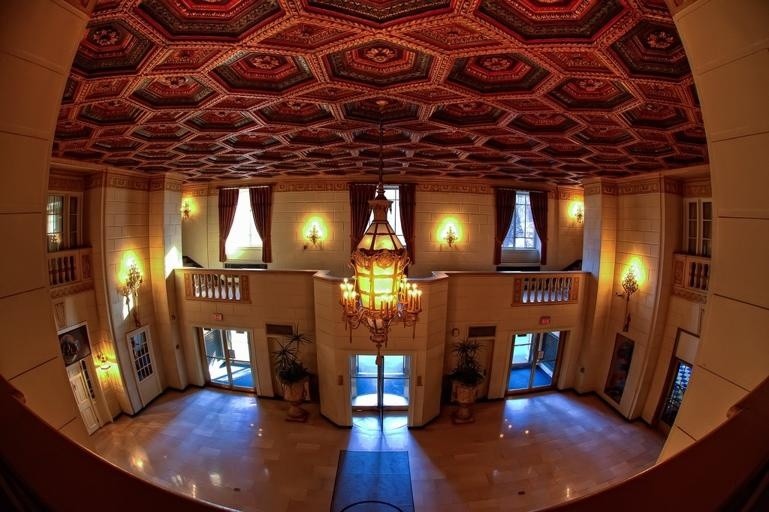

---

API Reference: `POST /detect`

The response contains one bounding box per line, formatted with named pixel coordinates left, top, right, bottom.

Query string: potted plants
left=442, top=334, right=490, bottom=425
left=269, top=317, right=315, bottom=425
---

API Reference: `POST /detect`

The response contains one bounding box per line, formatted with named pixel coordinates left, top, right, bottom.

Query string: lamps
left=567, top=195, right=584, bottom=225
left=95, top=349, right=112, bottom=380
left=115, top=246, right=145, bottom=328
left=435, top=214, right=464, bottom=248
left=180, top=193, right=200, bottom=226
left=613, top=245, right=649, bottom=332
left=337, top=116, right=424, bottom=371
left=300, top=213, right=328, bottom=252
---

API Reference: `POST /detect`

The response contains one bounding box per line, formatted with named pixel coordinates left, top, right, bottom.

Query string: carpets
left=329, top=448, right=416, bottom=511
